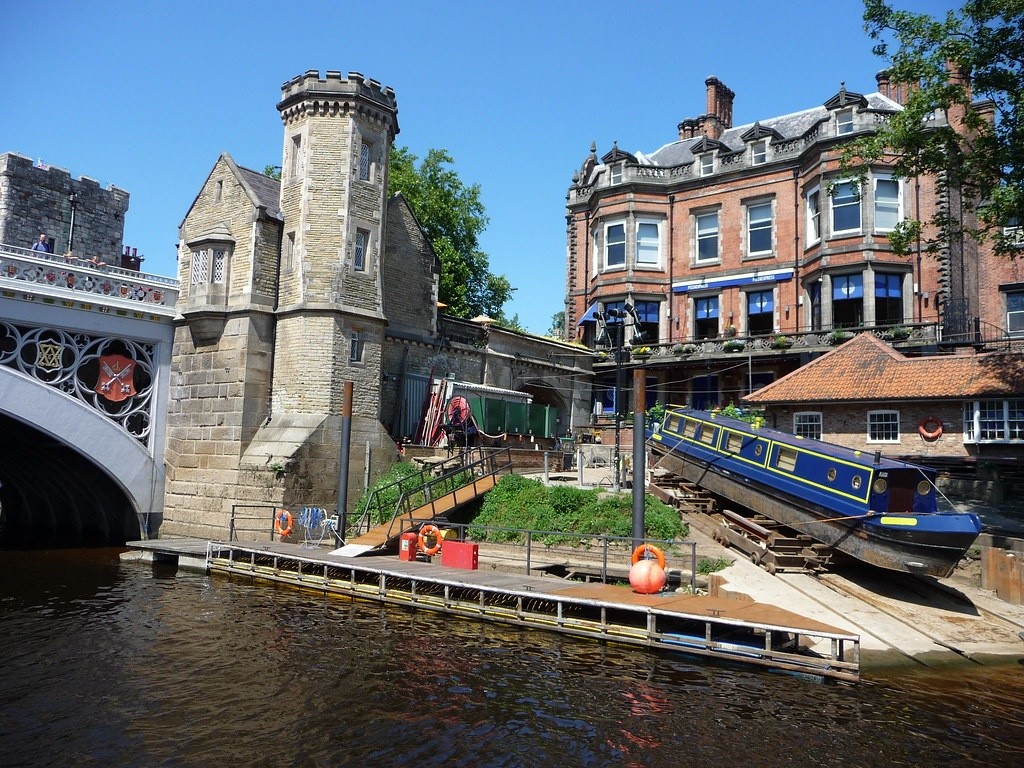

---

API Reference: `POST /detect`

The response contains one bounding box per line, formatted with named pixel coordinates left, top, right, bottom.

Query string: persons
left=63, top=251, right=79, bottom=288
left=86, top=255, right=106, bottom=293
left=31, top=233, right=50, bottom=283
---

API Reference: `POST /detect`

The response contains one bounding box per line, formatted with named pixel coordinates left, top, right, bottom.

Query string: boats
left=647, top=407, right=984, bottom=578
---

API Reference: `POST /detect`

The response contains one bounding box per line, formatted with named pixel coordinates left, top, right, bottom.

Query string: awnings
left=578, top=301, right=598, bottom=326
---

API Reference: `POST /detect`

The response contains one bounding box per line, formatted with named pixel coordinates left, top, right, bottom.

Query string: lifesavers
left=631, top=544, right=666, bottom=571
left=276, top=509, right=293, bottom=536
left=417, top=524, right=443, bottom=556
left=918, top=415, right=943, bottom=438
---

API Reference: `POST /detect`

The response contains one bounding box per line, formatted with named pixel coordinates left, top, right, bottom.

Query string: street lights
left=591, top=304, right=647, bottom=572
left=67, top=192, right=80, bottom=258
